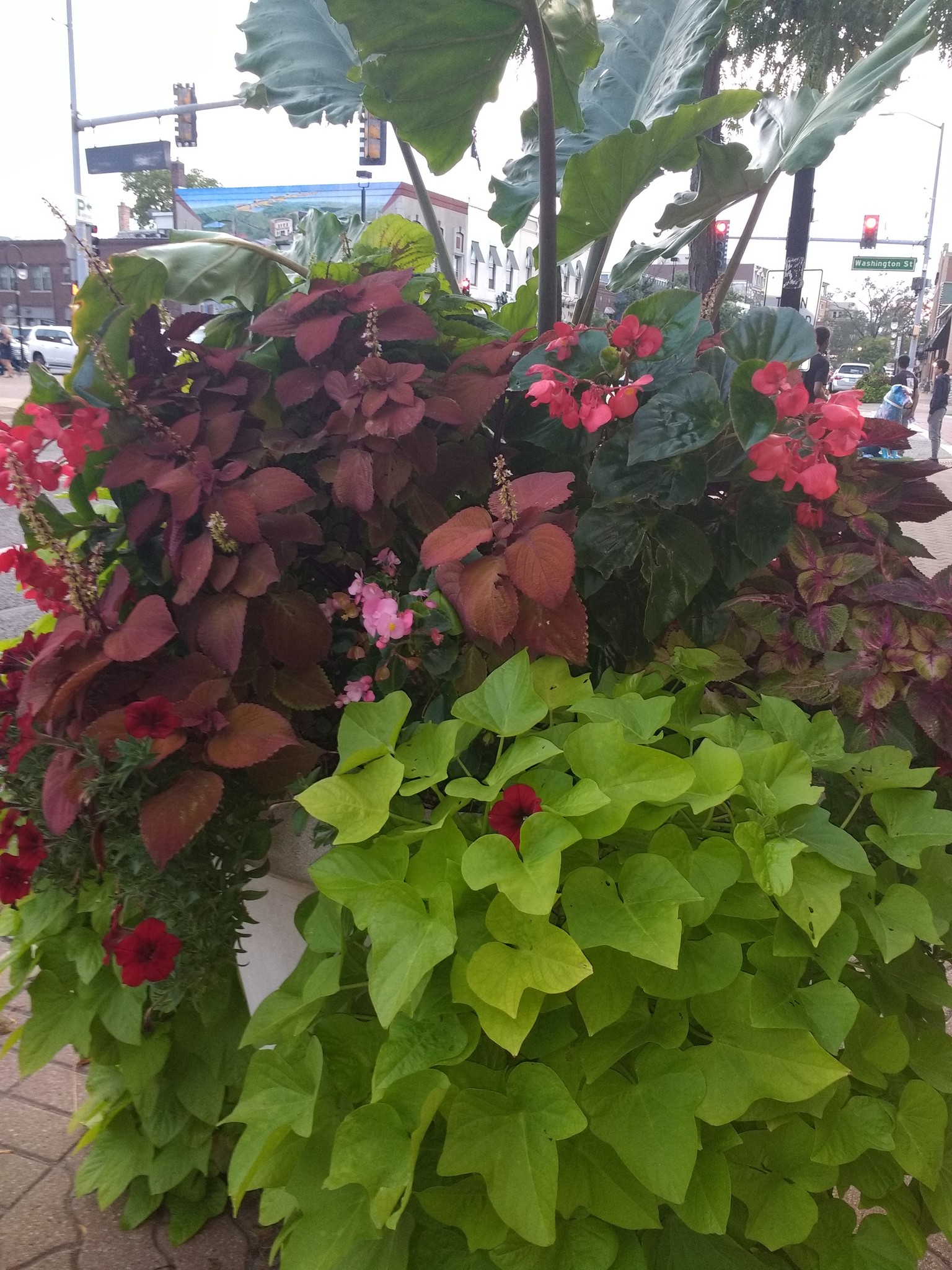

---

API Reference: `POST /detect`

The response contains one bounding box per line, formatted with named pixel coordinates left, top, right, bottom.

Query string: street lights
left=5, top=244, right=29, bottom=370
left=887, top=305, right=915, bottom=375
left=879, top=111, right=945, bottom=377
left=669, top=257, right=680, bottom=289
left=356, top=170, right=372, bottom=222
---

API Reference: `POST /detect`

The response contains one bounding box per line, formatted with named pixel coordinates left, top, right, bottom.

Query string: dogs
left=854, top=383, right=914, bottom=459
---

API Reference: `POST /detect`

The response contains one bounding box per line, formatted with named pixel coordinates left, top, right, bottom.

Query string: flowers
left=0, top=0, right=952, bottom=1269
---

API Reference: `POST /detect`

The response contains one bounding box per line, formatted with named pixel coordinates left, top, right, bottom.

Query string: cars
left=5, top=324, right=32, bottom=367
left=830, top=362, right=871, bottom=394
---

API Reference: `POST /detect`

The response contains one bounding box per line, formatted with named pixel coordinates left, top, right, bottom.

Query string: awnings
left=603, top=306, right=616, bottom=314
left=923, top=314, right=952, bottom=351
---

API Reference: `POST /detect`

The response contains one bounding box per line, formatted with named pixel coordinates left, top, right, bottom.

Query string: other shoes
left=928, top=457, right=939, bottom=464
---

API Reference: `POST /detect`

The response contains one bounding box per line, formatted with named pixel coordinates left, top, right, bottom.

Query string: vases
left=211, top=793, right=325, bottom=1013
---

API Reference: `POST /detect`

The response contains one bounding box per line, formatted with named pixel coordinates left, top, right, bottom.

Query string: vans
left=24, top=326, right=80, bottom=372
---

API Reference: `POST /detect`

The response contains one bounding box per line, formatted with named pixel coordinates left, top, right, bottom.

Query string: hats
left=898, top=354, right=910, bottom=364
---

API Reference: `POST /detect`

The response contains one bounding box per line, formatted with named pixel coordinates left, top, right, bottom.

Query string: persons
left=802, top=325, right=830, bottom=406
left=887, top=354, right=919, bottom=457
left=927, top=359, right=951, bottom=464
left=0, top=318, right=15, bottom=378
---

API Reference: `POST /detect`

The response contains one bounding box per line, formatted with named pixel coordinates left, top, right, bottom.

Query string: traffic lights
left=713, top=219, right=730, bottom=273
left=462, top=280, right=470, bottom=297
left=89, top=224, right=101, bottom=258
left=860, top=214, right=880, bottom=250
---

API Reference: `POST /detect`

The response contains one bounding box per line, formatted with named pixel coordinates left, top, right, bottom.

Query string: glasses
left=934, top=366, right=940, bottom=369
